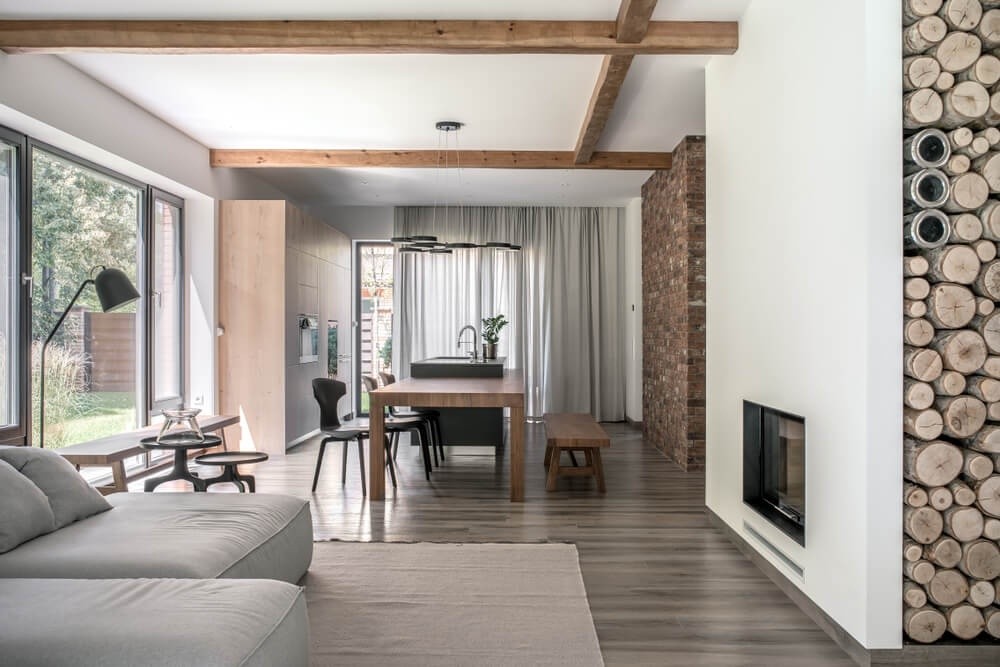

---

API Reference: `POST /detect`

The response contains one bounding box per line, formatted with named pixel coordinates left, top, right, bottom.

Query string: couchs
left=0, top=446, right=313, bottom=667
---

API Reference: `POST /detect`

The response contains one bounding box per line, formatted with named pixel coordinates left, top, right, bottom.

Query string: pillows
left=0, top=444, right=113, bottom=528
left=0, top=459, right=55, bottom=552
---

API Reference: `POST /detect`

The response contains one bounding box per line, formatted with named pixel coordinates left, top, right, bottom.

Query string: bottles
left=155, top=407, right=204, bottom=445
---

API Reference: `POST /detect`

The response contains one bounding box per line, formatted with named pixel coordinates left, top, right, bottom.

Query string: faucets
left=457, top=325, right=477, bottom=359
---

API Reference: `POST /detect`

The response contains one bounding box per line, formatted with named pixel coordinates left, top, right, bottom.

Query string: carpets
left=297, top=542, right=606, bottom=667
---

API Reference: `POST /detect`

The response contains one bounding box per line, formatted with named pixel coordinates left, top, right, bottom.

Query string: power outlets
left=194, top=396, right=203, bottom=405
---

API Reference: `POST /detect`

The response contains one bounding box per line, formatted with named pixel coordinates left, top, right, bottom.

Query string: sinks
left=437, top=356, right=474, bottom=364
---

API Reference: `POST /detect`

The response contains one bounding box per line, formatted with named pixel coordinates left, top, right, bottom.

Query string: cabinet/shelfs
left=218, top=200, right=351, bottom=457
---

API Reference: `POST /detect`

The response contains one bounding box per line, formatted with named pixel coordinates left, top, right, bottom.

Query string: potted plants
left=481, top=315, right=509, bottom=359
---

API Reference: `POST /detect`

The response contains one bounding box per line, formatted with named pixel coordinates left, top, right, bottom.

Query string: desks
left=369, top=368, right=525, bottom=502
left=195, top=451, right=269, bottom=492
left=142, top=434, right=222, bottom=492
left=56, top=415, right=240, bottom=496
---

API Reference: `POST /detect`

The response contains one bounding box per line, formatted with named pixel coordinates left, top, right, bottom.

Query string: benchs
left=544, top=412, right=611, bottom=494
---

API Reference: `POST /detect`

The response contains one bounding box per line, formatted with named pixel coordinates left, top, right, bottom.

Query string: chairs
left=312, top=371, right=445, bottom=496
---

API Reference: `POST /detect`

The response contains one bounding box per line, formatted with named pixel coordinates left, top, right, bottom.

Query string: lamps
left=41, top=265, right=140, bottom=448
left=391, top=122, right=521, bottom=254
